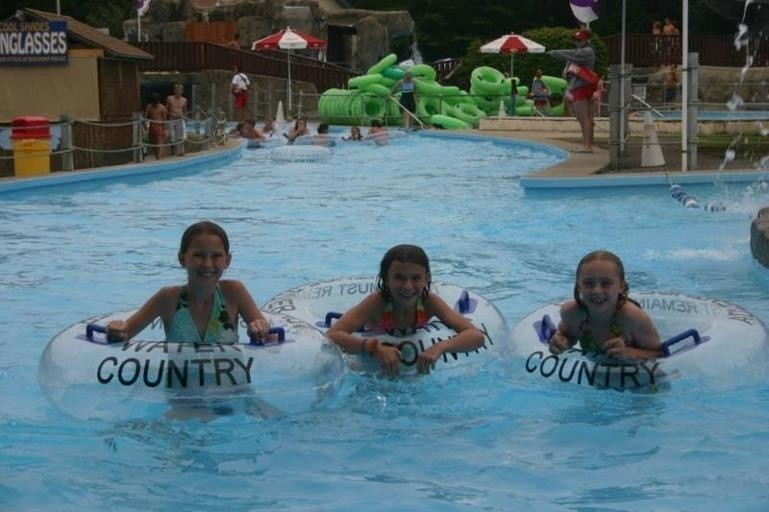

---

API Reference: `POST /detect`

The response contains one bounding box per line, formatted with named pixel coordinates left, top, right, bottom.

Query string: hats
left=570, top=31, right=591, bottom=41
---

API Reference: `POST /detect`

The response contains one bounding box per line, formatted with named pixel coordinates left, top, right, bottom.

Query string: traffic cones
left=497, top=99, right=508, bottom=118
left=274, top=100, right=287, bottom=124
left=640, top=110, right=666, bottom=168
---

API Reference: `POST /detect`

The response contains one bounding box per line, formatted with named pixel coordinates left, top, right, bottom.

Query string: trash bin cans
left=10, top=116, right=53, bottom=176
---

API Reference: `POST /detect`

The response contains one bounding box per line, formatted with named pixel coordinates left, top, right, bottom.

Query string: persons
left=531, top=68, right=551, bottom=113
left=227, top=118, right=392, bottom=148
left=108, top=221, right=286, bottom=424
left=593, top=76, right=606, bottom=118
left=392, top=70, right=419, bottom=130
left=324, top=244, right=485, bottom=376
left=165, top=83, right=188, bottom=157
left=227, top=33, right=241, bottom=51
left=231, top=64, right=251, bottom=120
left=550, top=249, right=664, bottom=361
left=143, top=92, right=166, bottom=161
left=653, top=17, right=680, bottom=102
left=548, top=30, right=599, bottom=153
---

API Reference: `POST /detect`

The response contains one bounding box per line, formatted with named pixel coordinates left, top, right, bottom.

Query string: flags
left=137, top=0, right=151, bottom=16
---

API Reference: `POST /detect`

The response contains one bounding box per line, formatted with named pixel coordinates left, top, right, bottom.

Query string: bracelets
left=370, top=339, right=379, bottom=356
left=361, top=338, right=368, bottom=354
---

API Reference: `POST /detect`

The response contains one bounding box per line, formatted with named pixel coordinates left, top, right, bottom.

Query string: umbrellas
left=480, top=31, right=546, bottom=78
left=251, top=25, right=326, bottom=111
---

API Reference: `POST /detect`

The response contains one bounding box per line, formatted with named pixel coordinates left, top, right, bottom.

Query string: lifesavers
left=508, top=290, right=767, bottom=393
left=36, top=308, right=345, bottom=428
left=260, top=276, right=507, bottom=377
left=317, top=53, right=567, bottom=129
